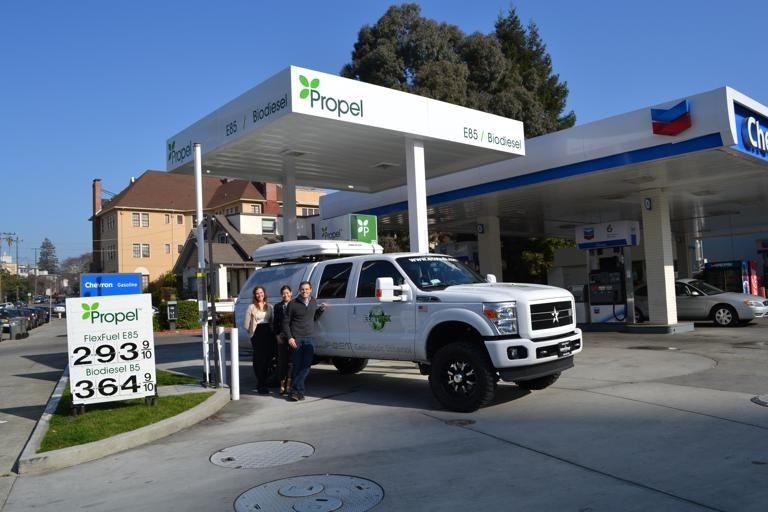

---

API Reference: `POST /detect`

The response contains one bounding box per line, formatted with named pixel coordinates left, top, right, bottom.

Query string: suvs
left=233, top=239, right=583, bottom=412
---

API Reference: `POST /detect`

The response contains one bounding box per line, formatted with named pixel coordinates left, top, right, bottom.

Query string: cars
left=633, top=278, right=768, bottom=328
left=0, top=295, right=66, bottom=330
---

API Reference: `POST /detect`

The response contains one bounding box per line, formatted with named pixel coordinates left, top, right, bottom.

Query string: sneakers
left=289, top=393, right=304, bottom=401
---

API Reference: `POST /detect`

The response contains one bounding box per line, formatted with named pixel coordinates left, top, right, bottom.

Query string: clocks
left=644, top=198, right=652, bottom=210
left=476, top=224, right=484, bottom=233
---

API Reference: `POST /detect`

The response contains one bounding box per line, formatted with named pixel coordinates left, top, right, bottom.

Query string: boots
left=279, top=378, right=292, bottom=394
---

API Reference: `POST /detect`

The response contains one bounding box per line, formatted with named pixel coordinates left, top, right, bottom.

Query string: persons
left=272, top=284, right=294, bottom=394
left=282, top=280, right=328, bottom=401
left=243, top=287, right=276, bottom=394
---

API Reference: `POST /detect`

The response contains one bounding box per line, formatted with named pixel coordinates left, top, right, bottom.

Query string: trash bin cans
left=8, top=317, right=29, bottom=340
